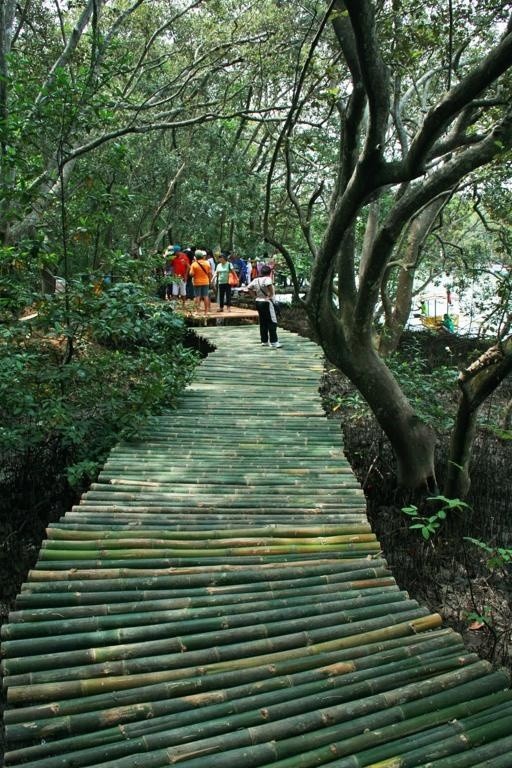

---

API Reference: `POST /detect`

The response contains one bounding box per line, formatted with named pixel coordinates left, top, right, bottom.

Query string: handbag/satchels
left=228, top=272, right=239, bottom=286
left=274, top=305, right=281, bottom=315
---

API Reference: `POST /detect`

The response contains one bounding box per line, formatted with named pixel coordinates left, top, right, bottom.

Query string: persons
left=157, top=241, right=239, bottom=316
left=415, top=299, right=427, bottom=319
left=435, top=313, right=454, bottom=335
left=246, top=265, right=284, bottom=348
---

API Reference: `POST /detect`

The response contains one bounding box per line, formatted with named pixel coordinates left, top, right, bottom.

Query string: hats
left=195, top=249, right=207, bottom=258
left=168, top=245, right=181, bottom=252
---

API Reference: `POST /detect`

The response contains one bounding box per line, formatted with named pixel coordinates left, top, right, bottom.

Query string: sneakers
left=262, top=342, right=283, bottom=348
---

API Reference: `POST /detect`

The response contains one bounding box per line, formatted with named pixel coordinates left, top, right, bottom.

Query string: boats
left=414, top=313, right=459, bottom=336
